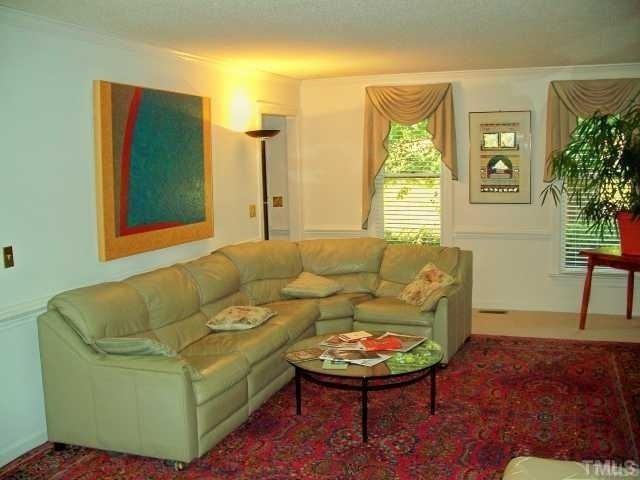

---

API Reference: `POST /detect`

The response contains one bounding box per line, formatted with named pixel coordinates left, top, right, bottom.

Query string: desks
left=579, top=248, right=640, bottom=329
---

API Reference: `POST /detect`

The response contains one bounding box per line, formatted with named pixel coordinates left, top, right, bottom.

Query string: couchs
left=37, top=237, right=473, bottom=472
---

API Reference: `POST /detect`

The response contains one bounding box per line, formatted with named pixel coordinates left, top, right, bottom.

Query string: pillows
left=205, top=305, right=278, bottom=331
left=280, top=271, right=344, bottom=297
left=396, top=261, right=456, bottom=307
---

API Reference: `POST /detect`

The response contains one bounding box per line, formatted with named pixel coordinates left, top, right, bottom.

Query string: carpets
left=0, top=334, right=640, bottom=480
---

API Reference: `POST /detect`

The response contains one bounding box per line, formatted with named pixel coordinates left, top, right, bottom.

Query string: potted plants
left=538, top=91, right=640, bottom=256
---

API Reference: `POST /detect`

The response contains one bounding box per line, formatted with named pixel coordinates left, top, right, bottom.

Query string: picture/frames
left=469, top=110, right=532, bottom=204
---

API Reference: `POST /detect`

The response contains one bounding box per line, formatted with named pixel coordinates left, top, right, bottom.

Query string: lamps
left=245, top=130, right=282, bottom=241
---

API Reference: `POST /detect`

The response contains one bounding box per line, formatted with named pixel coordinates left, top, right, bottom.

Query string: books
left=283, top=330, right=429, bottom=370
